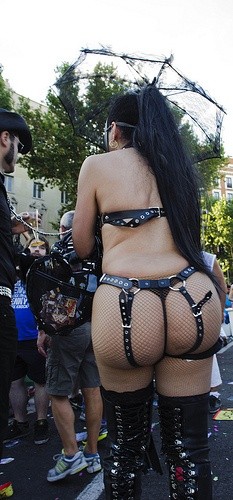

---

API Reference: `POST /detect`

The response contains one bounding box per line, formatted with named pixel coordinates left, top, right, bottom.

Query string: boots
left=156, top=392, right=214, bottom=500
left=100, top=379, right=154, bottom=500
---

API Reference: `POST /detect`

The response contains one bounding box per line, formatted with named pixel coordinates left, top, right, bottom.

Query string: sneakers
left=80, top=441, right=102, bottom=474
left=46, top=448, right=88, bottom=482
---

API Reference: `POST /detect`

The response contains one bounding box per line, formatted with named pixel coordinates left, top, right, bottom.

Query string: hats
left=0, top=108, right=32, bottom=155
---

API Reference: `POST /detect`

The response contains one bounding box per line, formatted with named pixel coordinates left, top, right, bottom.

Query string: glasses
left=13, top=135, right=24, bottom=153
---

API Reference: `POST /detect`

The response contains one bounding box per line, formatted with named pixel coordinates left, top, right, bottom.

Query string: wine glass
left=26, top=208, right=45, bottom=246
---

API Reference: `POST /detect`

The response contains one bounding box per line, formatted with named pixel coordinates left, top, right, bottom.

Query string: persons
left=0, top=84, right=233, bottom=500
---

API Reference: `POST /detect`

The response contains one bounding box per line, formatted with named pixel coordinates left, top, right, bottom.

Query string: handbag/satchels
left=26, top=231, right=103, bottom=338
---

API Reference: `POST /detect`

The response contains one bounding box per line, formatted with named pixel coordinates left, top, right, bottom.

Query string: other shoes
left=2, top=418, right=32, bottom=444
left=33, top=419, right=50, bottom=444
left=208, top=395, right=222, bottom=413
left=79, top=412, right=108, bottom=445
left=69, top=393, right=82, bottom=410
